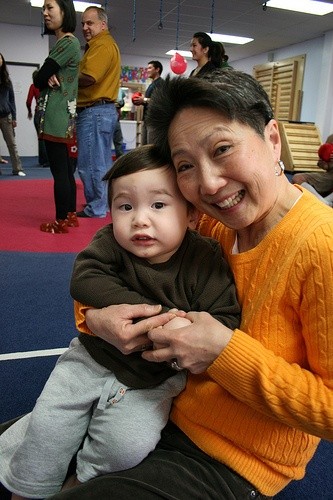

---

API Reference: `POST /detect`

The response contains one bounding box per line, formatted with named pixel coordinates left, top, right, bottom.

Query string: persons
left=50, top=69, right=333, bottom=500
left=33, top=0, right=79, bottom=234
left=0, top=54, right=50, bottom=177
left=134, top=60, right=167, bottom=146
left=291, top=134, right=333, bottom=196
left=112, top=89, right=125, bottom=159
left=188, top=32, right=232, bottom=79
left=76, top=6, right=121, bottom=218
left=0, top=145, right=241, bottom=500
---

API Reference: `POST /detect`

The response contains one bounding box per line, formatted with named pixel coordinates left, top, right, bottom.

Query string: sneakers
left=40, top=219, right=69, bottom=234
left=65, top=213, right=79, bottom=227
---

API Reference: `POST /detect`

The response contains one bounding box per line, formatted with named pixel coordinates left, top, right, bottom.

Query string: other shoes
left=12, top=171, right=26, bottom=177
left=76, top=211, right=106, bottom=219
left=112, top=154, right=117, bottom=161
left=0, top=159, right=8, bottom=164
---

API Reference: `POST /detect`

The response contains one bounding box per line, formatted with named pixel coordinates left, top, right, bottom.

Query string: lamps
left=29, top=0, right=104, bottom=12
left=166, top=0, right=333, bottom=58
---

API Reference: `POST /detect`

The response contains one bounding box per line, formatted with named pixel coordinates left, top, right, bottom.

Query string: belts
left=75, top=99, right=115, bottom=114
